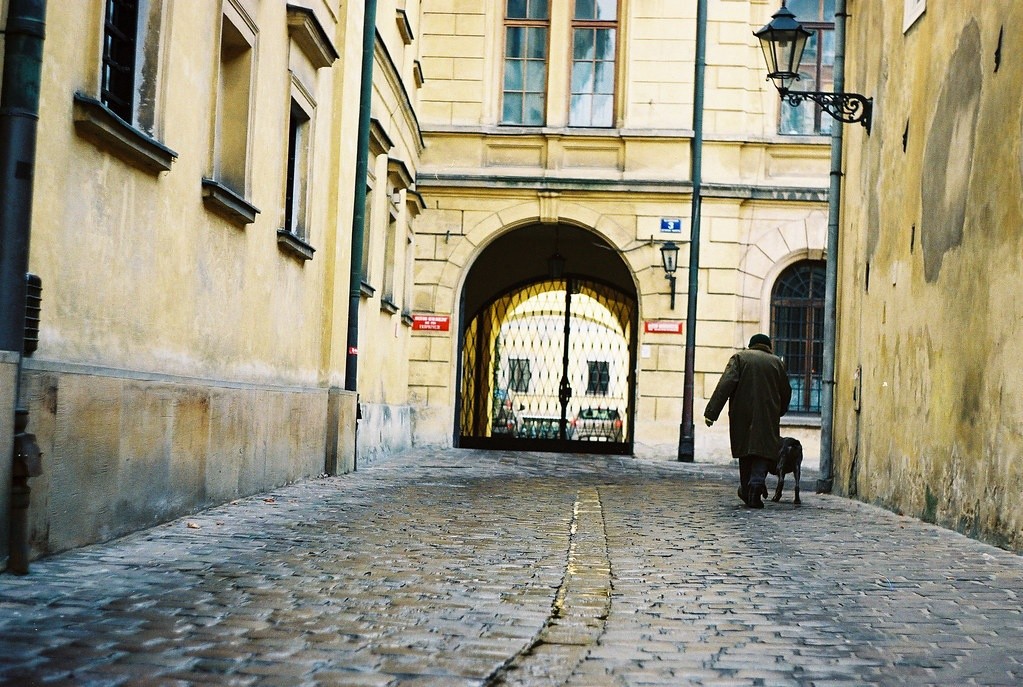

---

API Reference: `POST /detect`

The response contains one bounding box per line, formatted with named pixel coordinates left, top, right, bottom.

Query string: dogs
left=761, top=436, right=803, bottom=504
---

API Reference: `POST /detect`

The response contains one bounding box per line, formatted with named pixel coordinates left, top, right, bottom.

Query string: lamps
left=752, top=1, right=874, bottom=138
left=660, top=240, right=680, bottom=312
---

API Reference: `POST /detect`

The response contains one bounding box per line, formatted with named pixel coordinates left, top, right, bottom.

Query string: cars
left=519, top=415, right=572, bottom=440
left=572, top=404, right=624, bottom=443
left=492, top=385, right=519, bottom=437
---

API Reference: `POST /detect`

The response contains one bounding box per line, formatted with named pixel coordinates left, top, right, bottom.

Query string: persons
left=703, top=333, right=792, bottom=509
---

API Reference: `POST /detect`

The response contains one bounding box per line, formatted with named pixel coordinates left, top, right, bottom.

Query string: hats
left=749, top=334, right=771, bottom=349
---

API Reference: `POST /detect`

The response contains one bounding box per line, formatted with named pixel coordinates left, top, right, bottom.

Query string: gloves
left=705, top=416, right=713, bottom=427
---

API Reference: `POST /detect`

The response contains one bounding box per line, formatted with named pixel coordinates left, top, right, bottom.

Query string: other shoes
left=738, top=485, right=748, bottom=503
left=751, top=496, right=764, bottom=508
